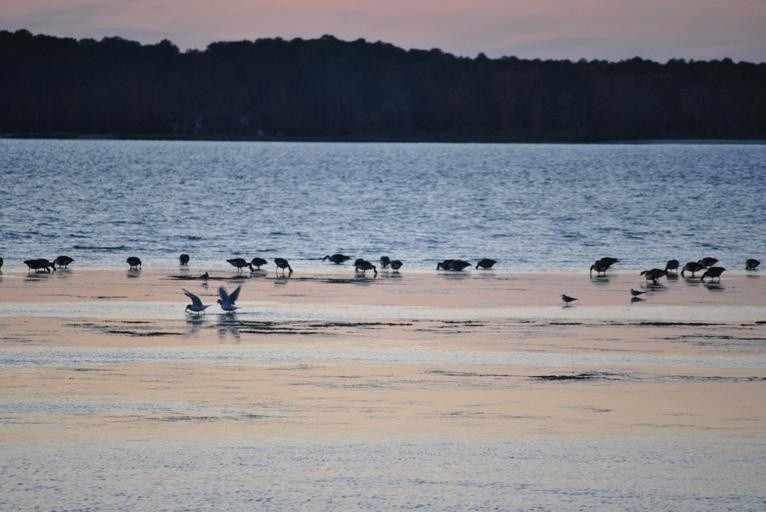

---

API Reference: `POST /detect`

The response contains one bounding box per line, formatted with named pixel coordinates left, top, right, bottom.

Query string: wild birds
left=746, top=259, right=760, bottom=269
left=631, top=258, right=726, bottom=296
left=590, top=261, right=610, bottom=277
left=182, top=289, right=212, bottom=315
left=601, top=258, right=619, bottom=266
left=180, top=254, right=190, bottom=265
left=0, top=258, right=3, bottom=268
left=561, top=294, right=578, bottom=307
left=126, top=257, right=141, bottom=270
left=226, top=254, right=497, bottom=280
left=24, top=256, right=75, bottom=276
left=217, top=286, right=241, bottom=313
